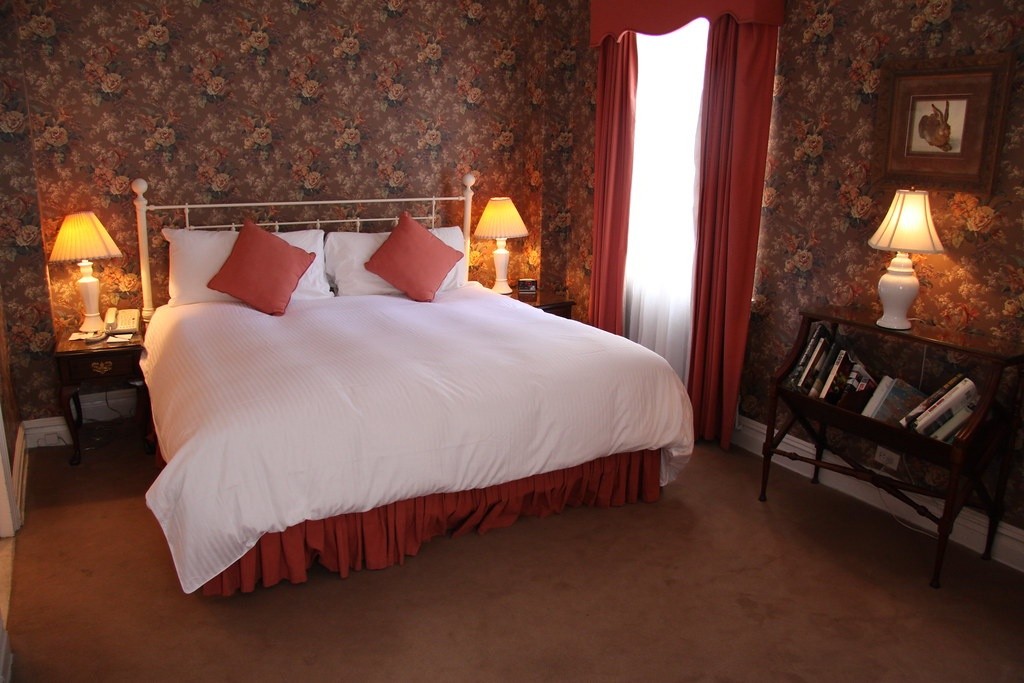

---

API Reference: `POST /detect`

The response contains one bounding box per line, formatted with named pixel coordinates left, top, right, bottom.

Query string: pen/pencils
left=111, top=335, right=131, bottom=340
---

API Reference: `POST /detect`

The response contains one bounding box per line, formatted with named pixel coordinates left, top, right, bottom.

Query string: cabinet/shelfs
left=758, top=305, right=1024, bottom=588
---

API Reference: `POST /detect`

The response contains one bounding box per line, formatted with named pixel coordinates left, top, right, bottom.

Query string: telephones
left=104, top=307, right=140, bottom=335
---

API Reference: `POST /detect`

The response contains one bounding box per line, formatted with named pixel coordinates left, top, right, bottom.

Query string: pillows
left=162, top=227, right=334, bottom=307
left=364, top=211, right=464, bottom=302
left=207, top=219, right=316, bottom=316
left=324, top=225, right=468, bottom=296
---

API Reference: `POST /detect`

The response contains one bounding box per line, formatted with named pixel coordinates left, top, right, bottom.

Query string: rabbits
left=919, top=100, right=952, bottom=151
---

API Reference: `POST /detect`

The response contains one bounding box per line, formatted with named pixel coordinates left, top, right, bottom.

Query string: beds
left=132, top=173, right=694, bottom=596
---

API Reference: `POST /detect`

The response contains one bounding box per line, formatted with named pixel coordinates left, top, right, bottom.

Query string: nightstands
left=504, top=288, right=576, bottom=320
left=53, top=328, right=150, bottom=466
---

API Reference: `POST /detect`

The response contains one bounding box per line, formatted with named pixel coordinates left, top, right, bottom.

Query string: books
left=785, top=323, right=982, bottom=446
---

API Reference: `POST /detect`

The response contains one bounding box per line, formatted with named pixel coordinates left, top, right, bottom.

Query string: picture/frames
left=887, top=71, right=995, bottom=185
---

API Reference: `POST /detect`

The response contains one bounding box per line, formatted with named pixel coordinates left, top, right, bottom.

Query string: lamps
left=49, top=210, right=122, bottom=333
left=473, top=196, right=530, bottom=295
left=868, top=185, right=944, bottom=329
